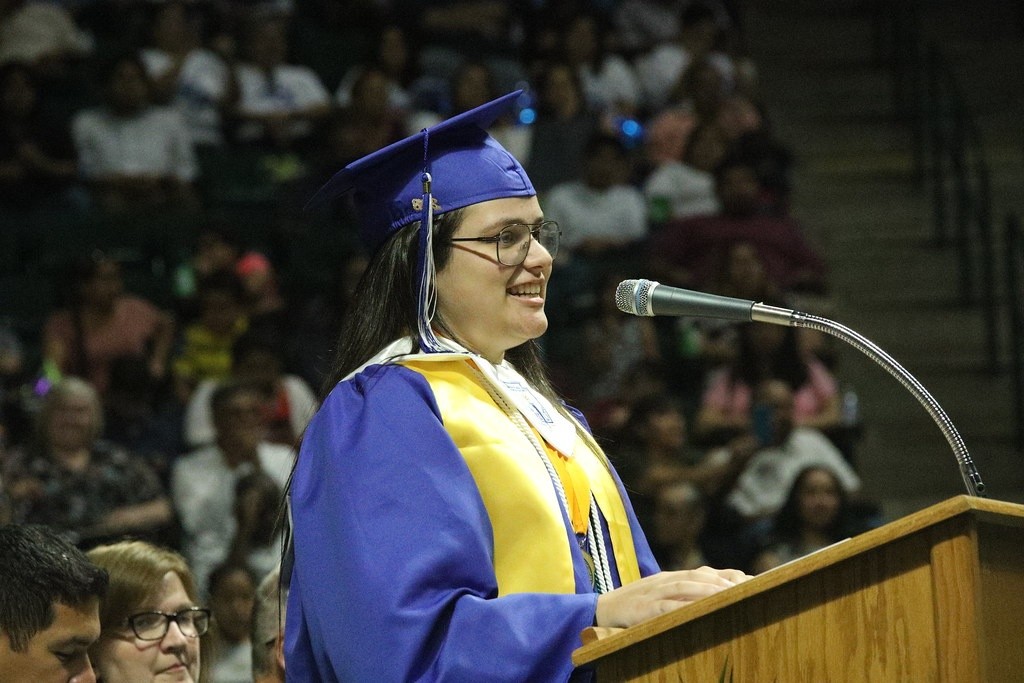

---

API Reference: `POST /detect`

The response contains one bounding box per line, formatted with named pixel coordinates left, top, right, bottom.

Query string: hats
left=301, top=89, right=537, bottom=353
left=232, top=253, right=274, bottom=277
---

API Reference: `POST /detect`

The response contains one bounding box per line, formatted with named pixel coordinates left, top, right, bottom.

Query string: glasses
left=117, top=607, right=215, bottom=642
left=224, top=408, right=279, bottom=423
left=450, top=219, right=565, bottom=269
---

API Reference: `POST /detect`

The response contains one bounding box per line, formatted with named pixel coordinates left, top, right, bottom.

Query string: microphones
left=616, top=280, right=797, bottom=325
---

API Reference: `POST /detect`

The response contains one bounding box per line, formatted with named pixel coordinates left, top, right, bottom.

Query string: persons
left=282, top=89, right=753, bottom=682
left=0, top=0, right=864, bottom=683
